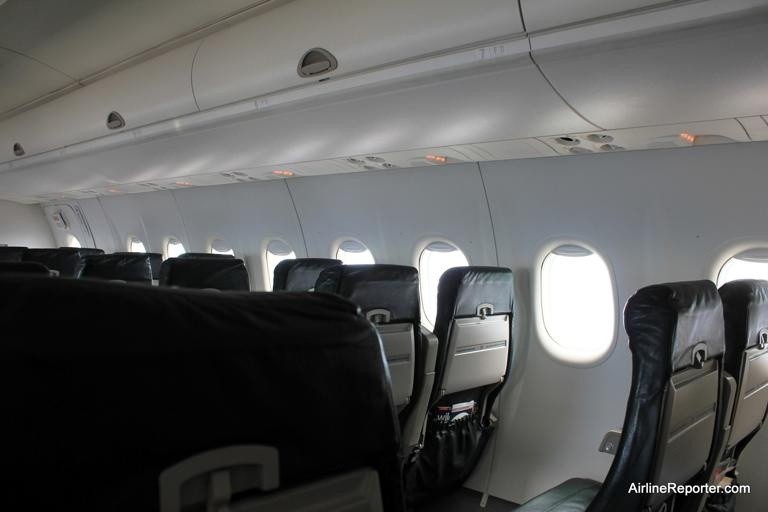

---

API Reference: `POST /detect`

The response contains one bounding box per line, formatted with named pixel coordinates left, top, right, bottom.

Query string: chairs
left=0, top=241, right=767, bottom=512
